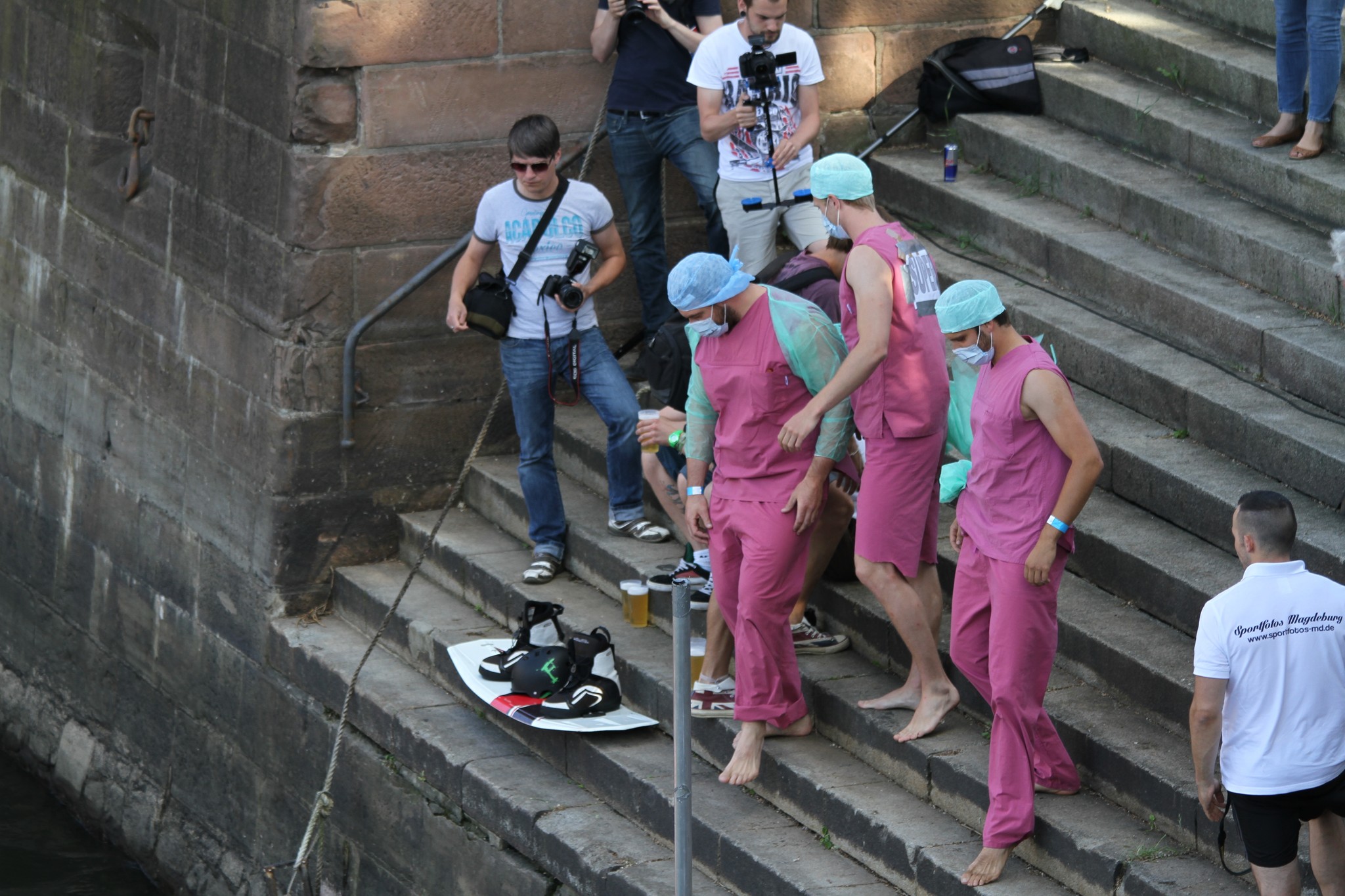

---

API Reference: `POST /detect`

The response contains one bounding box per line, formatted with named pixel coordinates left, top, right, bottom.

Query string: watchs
left=669, top=430, right=684, bottom=446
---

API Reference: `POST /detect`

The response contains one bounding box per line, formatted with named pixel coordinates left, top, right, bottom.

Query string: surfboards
left=448, top=594, right=664, bottom=733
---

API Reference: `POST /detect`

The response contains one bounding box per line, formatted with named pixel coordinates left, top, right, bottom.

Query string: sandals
left=1289, top=140, right=1323, bottom=160
left=1252, top=130, right=1297, bottom=149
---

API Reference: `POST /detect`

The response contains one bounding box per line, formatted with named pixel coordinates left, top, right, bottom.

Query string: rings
left=450, top=326, right=454, bottom=330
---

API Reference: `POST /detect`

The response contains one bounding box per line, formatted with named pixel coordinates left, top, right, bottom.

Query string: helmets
left=512, top=646, right=570, bottom=698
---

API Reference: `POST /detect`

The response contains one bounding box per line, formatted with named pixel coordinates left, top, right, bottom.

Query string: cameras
left=542, top=239, right=601, bottom=309
left=739, top=35, right=797, bottom=88
left=623, top=0, right=650, bottom=25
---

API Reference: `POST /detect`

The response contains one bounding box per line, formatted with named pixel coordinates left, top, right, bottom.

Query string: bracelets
left=676, top=434, right=687, bottom=453
left=686, top=487, right=704, bottom=497
left=1047, top=515, right=1069, bottom=533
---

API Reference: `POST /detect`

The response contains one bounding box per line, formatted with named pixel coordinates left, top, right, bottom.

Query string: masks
left=689, top=305, right=729, bottom=338
left=822, top=198, right=851, bottom=239
left=951, top=326, right=994, bottom=366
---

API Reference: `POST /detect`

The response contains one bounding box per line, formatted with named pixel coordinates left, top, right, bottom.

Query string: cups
left=627, top=585, right=649, bottom=628
left=638, top=409, right=660, bottom=453
left=691, top=637, right=706, bottom=691
left=619, top=580, right=642, bottom=622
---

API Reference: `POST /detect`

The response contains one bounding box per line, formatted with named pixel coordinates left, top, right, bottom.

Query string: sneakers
left=608, top=515, right=671, bottom=542
left=646, top=558, right=711, bottom=592
left=690, top=674, right=737, bottom=718
left=541, top=626, right=622, bottom=719
left=788, top=616, right=849, bottom=655
left=522, top=552, right=565, bottom=585
left=478, top=600, right=567, bottom=682
left=690, top=572, right=713, bottom=610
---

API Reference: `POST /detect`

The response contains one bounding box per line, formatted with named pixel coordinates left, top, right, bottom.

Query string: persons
left=1188, top=491, right=1345, bottom=896
left=589, top=0, right=726, bottom=382
left=934, top=277, right=1106, bottom=887
left=685, top=0, right=828, bottom=280
left=1251, top=0, right=1345, bottom=160
left=446, top=114, right=671, bottom=585
left=668, top=153, right=977, bottom=786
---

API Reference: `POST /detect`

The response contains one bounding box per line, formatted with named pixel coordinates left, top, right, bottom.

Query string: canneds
left=944, top=144, right=958, bottom=182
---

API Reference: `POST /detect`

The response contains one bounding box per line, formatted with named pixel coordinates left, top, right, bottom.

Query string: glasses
left=508, top=155, right=554, bottom=172
